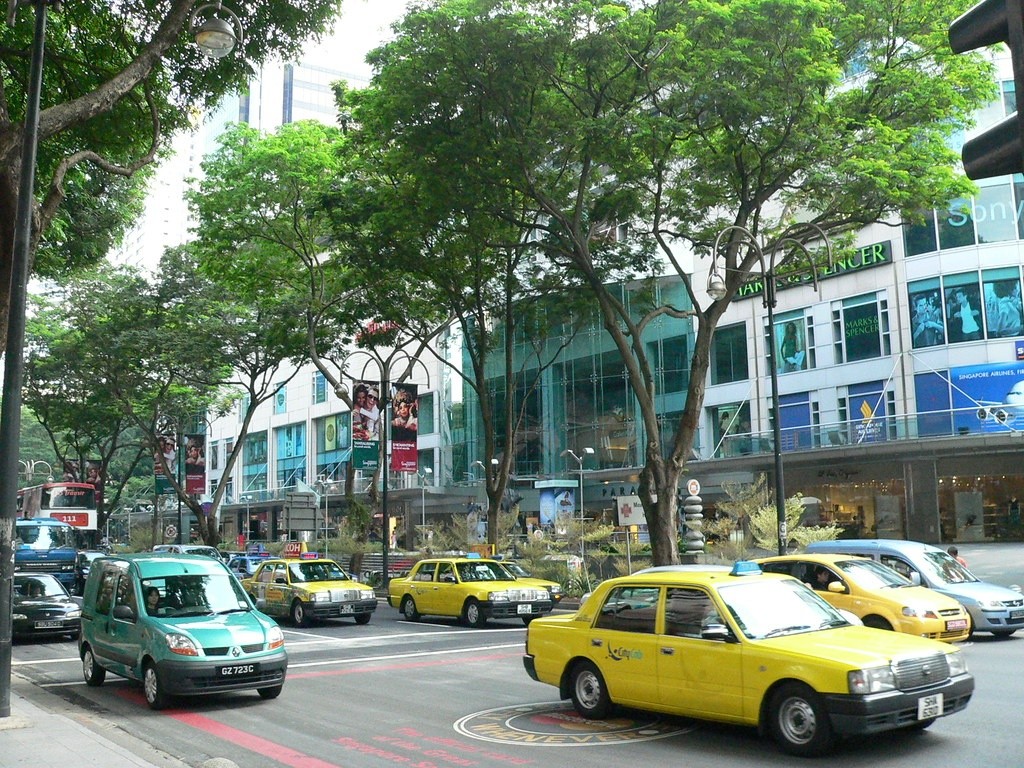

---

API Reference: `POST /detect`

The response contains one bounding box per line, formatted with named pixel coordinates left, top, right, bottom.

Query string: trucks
left=14, top=517, right=78, bottom=596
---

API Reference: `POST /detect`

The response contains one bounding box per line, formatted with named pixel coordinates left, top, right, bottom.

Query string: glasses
left=165, top=442, right=174, bottom=446
left=367, top=395, right=378, bottom=401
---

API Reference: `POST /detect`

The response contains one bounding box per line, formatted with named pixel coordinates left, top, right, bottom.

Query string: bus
left=19, top=482, right=98, bottom=553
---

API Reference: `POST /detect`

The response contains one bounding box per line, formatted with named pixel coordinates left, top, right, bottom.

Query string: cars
left=82, top=552, right=287, bottom=710
left=388, top=552, right=554, bottom=627
left=11, top=572, right=83, bottom=645
left=489, top=554, right=564, bottom=608
left=152, top=542, right=283, bottom=582
left=577, top=564, right=864, bottom=627
left=805, top=540, right=1023, bottom=638
left=520, top=559, right=976, bottom=757
left=743, top=553, right=971, bottom=643
left=239, top=551, right=377, bottom=628
left=75, top=548, right=107, bottom=594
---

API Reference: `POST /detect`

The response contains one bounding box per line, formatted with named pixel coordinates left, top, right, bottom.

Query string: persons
left=392, top=531, right=397, bottom=549
left=912, top=294, right=944, bottom=347
left=354, top=386, right=380, bottom=433
left=86, top=469, right=101, bottom=484
left=943, top=546, right=967, bottom=579
left=144, top=586, right=165, bottom=619
left=354, top=384, right=368, bottom=428
left=185, top=445, right=205, bottom=466
left=68, top=462, right=79, bottom=480
left=992, top=279, right=1024, bottom=337
left=1008, top=496, right=1021, bottom=537
left=780, top=323, right=801, bottom=374
left=952, top=290, right=982, bottom=341
left=676, top=596, right=721, bottom=638
left=163, top=437, right=176, bottom=469
left=810, top=566, right=833, bottom=591
left=895, top=561, right=909, bottom=577
left=392, top=400, right=418, bottom=431
left=154, top=437, right=165, bottom=463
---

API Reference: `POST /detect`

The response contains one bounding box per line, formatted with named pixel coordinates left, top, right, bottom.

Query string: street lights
left=470, top=456, right=499, bottom=523
left=104, top=510, right=113, bottom=546
left=238, top=495, right=251, bottom=546
left=0, top=0, right=244, bottom=724
left=123, top=508, right=133, bottom=543
left=706, top=221, right=835, bottom=556
left=407, top=464, right=434, bottom=548
left=141, top=413, right=212, bottom=545
left=313, top=479, right=334, bottom=559
left=333, top=347, right=433, bottom=592
left=560, top=447, right=596, bottom=562
